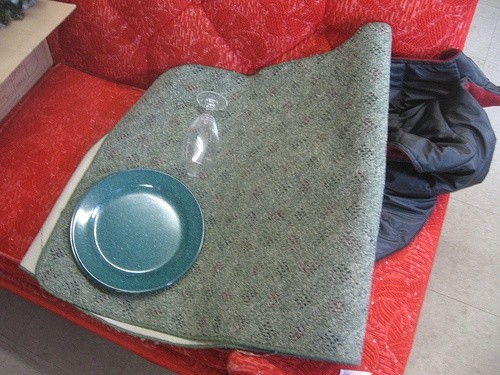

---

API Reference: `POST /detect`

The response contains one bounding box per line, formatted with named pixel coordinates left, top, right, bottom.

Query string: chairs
left=1, top=1, right=478, bottom=375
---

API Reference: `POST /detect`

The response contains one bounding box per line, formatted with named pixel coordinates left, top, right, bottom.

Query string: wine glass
left=182, top=91, right=228, bottom=177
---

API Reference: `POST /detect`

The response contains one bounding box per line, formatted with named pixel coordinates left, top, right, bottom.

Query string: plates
left=69, top=169, right=205, bottom=293
